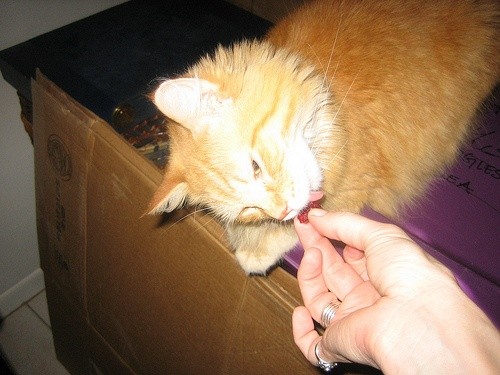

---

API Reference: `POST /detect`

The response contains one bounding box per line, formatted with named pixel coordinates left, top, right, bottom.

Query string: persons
left=290, top=209, right=500, bottom=374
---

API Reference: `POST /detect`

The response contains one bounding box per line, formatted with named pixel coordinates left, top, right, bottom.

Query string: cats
left=138, top=0, right=500, bottom=370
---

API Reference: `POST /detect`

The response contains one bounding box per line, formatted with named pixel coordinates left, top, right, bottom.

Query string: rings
left=321, top=297, right=341, bottom=328
left=310, top=340, right=337, bottom=373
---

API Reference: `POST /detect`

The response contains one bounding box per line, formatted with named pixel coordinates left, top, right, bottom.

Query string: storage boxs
left=31, top=2, right=499, bottom=374
